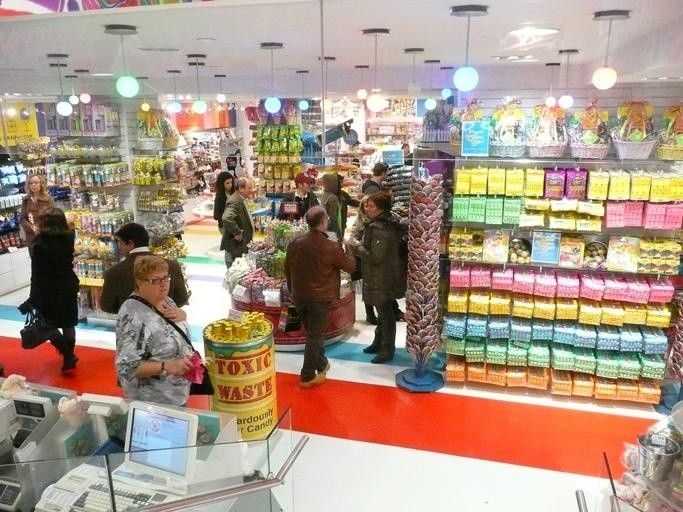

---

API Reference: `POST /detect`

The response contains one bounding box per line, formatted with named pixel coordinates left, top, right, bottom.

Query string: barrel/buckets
left=203, top=317, right=279, bottom=442
left=637, top=433, right=680, bottom=482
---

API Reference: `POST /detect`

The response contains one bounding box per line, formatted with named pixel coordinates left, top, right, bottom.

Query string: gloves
left=17, top=301, right=34, bottom=314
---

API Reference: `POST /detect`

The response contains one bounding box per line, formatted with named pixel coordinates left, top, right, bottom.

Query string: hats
left=295, top=173, right=315, bottom=183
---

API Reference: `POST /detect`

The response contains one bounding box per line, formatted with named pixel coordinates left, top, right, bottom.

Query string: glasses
left=142, top=275, right=173, bottom=284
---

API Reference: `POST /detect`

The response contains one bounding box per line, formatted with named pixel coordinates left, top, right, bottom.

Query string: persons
left=275, top=161, right=407, bottom=388
left=401, top=143, right=413, bottom=166
left=111, top=255, right=216, bottom=409
left=17, top=173, right=53, bottom=260
left=219, top=177, right=253, bottom=269
left=98, top=223, right=186, bottom=386
left=16, top=206, right=79, bottom=374
left=213, top=171, right=235, bottom=236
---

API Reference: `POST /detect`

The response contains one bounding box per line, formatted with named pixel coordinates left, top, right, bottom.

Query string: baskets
left=614, top=140, right=655, bottom=159
left=491, top=140, right=525, bottom=158
left=138, top=136, right=180, bottom=150
left=657, top=143, right=683, bottom=161
left=570, top=143, right=608, bottom=159
left=527, top=141, right=567, bottom=158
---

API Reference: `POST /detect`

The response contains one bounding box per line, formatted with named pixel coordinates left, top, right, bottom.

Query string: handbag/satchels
left=351, top=255, right=363, bottom=282
left=20, top=321, right=50, bottom=349
left=189, top=364, right=214, bottom=395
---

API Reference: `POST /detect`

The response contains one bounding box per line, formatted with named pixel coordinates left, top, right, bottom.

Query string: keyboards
left=70, top=477, right=177, bottom=512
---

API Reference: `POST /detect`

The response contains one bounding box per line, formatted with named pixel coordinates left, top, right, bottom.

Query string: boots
left=50, top=334, right=79, bottom=372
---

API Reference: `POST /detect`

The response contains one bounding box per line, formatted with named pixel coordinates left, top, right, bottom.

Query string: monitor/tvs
left=123, top=401, right=197, bottom=480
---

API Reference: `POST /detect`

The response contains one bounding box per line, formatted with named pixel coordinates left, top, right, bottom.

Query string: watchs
left=159, top=361, right=166, bottom=378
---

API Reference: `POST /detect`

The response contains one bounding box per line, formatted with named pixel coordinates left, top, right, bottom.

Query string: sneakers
left=371, top=355, right=394, bottom=363
left=396, top=312, right=406, bottom=321
left=316, top=363, right=330, bottom=374
left=363, top=344, right=381, bottom=353
left=367, top=316, right=378, bottom=325
left=299, top=373, right=325, bottom=389
